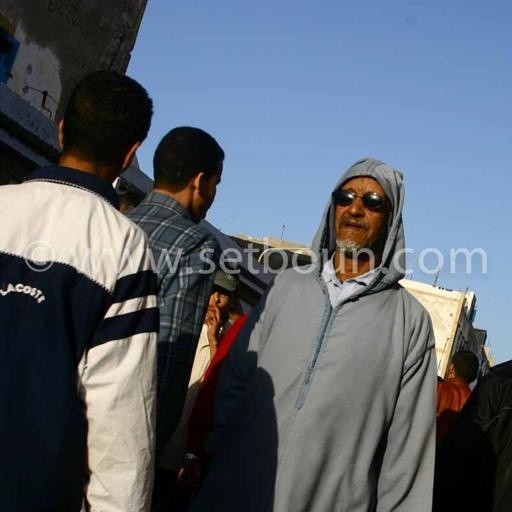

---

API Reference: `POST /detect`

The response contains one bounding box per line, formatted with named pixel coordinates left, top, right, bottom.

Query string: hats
left=213, top=265, right=240, bottom=291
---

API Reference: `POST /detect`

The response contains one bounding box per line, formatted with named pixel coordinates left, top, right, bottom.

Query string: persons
left=189, top=157, right=436, bottom=512
left=150, top=269, right=248, bottom=512
left=0, top=70, right=160, bottom=511
left=437, top=350, right=480, bottom=417
left=432, top=360, right=512, bottom=512
left=123, top=128, right=225, bottom=469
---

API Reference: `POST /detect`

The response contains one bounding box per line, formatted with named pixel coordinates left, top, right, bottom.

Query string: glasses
left=333, top=189, right=386, bottom=210
